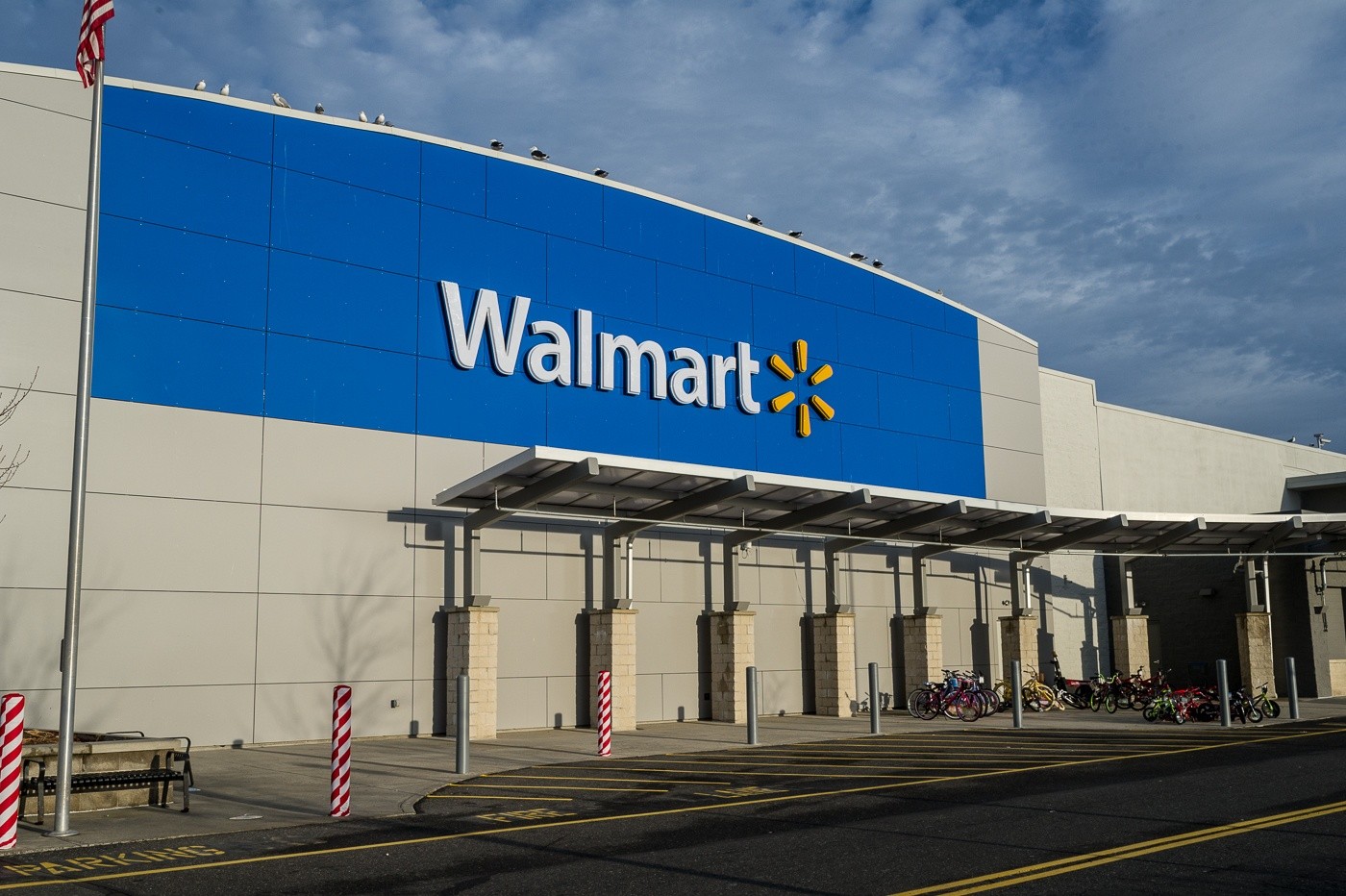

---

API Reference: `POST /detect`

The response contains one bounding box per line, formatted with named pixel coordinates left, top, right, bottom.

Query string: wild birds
left=1288, top=435, right=1297, bottom=443
left=784, top=230, right=805, bottom=239
left=746, top=214, right=764, bottom=226
left=529, top=147, right=551, bottom=162
left=373, top=112, right=395, bottom=127
left=490, top=138, right=505, bottom=153
left=937, top=289, right=944, bottom=297
left=593, top=168, right=610, bottom=178
left=357, top=109, right=369, bottom=123
left=193, top=80, right=207, bottom=92
left=873, top=258, right=886, bottom=269
left=849, top=251, right=868, bottom=262
left=272, top=93, right=292, bottom=108
left=315, top=102, right=324, bottom=115
left=220, top=84, right=231, bottom=96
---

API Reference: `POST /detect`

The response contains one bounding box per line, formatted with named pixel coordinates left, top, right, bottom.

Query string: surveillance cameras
left=1320, top=439, right=1331, bottom=443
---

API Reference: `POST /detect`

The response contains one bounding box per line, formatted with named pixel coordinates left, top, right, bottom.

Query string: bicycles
left=906, top=651, right=1281, bottom=725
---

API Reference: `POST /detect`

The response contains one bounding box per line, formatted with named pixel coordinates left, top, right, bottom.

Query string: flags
left=76, top=0, right=115, bottom=89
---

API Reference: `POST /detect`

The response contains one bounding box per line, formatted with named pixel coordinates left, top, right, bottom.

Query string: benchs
left=17, top=751, right=189, bottom=825
